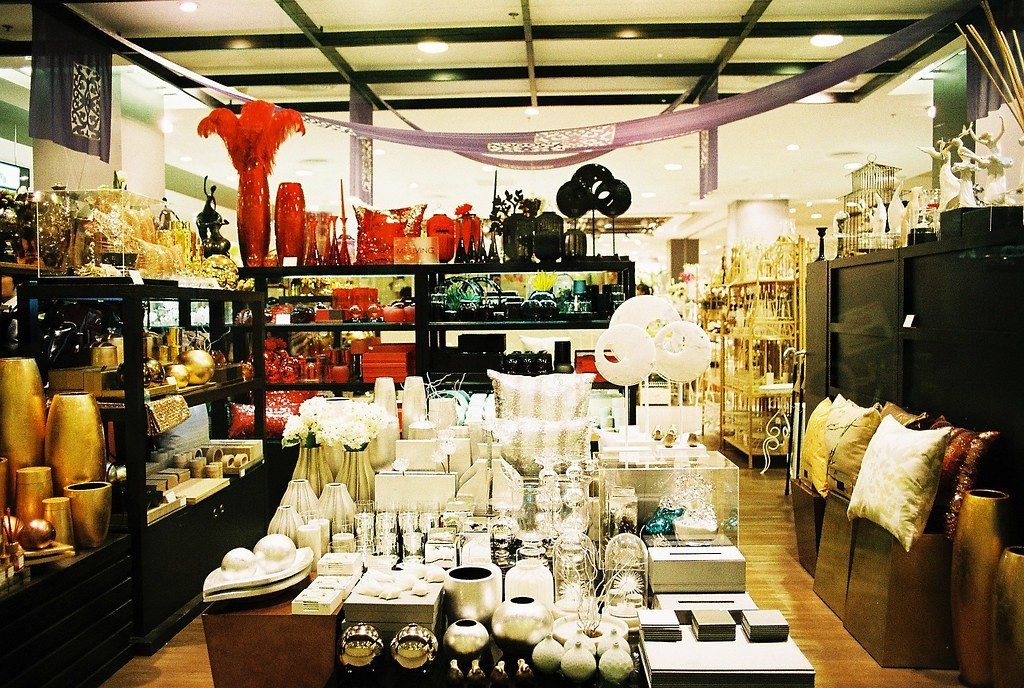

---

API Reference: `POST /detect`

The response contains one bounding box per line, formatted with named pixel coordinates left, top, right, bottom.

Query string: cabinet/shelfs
left=17, top=277, right=271, bottom=654
left=1, top=527, right=133, bottom=688
left=211, top=256, right=635, bottom=520
left=703, top=239, right=819, bottom=470
left=806, top=238, right=1024, bottom=542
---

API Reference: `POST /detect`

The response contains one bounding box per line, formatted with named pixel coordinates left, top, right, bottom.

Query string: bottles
left=1, top=180, right=973, bottom=686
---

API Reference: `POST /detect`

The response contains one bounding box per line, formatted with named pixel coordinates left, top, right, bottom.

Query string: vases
left=427, top=213, right=485, bottom=262
left=237, top=169, right=350, bottom=265
left=267, top=374, right=427, bottom=550
left=504, top=212, right=586, bottom=263
left=950, top=487, right=1024, bottom=688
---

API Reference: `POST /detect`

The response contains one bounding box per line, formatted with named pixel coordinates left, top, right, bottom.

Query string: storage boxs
left=431, top=333, right=504, bottom=380
left=594, top=450, right=759, bottom=625
left=363, top=343, right=416, bottom=385
left=291, top=558, right=364, bottom=614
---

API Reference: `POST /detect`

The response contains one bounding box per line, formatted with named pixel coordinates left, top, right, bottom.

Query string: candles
left=299, top=518, right=354, bottom=573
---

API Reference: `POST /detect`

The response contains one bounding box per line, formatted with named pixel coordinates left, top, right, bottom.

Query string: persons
left=952, top=138, right=982, bottom=207
left=970, top=114, right=1006, bottom=204
left=637, top=283, right=654, bottom=295
left=936, top=122, right=973, bottom=209
left=159, top=197, right=179, bottom=229
left=203, top=175, right=217, bottom=212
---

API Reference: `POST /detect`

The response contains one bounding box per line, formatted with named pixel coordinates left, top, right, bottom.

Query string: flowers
left=455, top=203, right=473, bottom=217
left=280, top=397, right=344, bottom=452
left=315, top=401, right=389, bottom=452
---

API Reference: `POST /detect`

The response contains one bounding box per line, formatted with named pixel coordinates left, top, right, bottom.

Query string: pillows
left=485, top=369, right=598, bottom=475
left=801, top=393, right=1000, bottom=552
left=350, top=202, right=428, bottom=264
